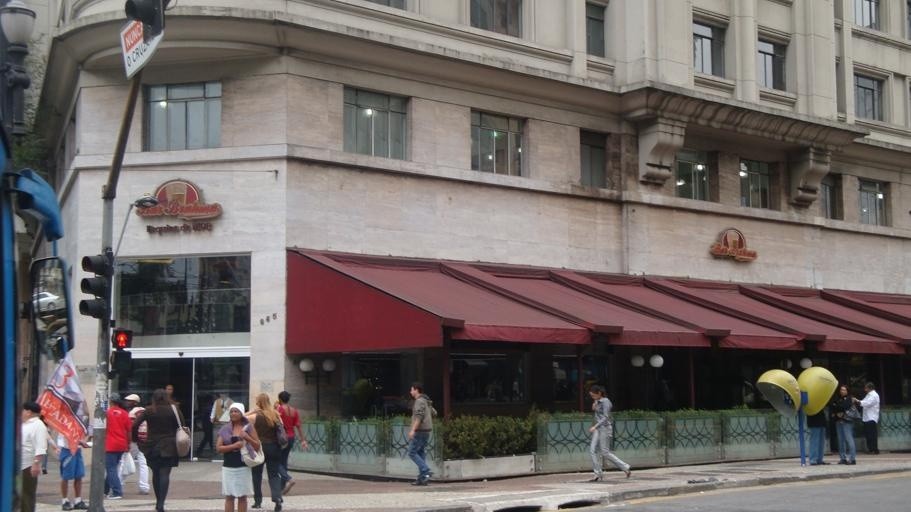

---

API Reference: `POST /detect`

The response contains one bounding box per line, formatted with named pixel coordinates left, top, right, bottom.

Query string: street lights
left=1, top=0, right=38, bottom=171
left=782, top=356, right=814, bottom=378
left=114, top=194, right=160, bottom=263
left=632, top=353, right=666, bottom=411
left=300, top=355, right=338, bottom=418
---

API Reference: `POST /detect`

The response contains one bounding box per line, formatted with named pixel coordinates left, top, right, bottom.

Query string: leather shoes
left=810, top=450, right=881, bottom=465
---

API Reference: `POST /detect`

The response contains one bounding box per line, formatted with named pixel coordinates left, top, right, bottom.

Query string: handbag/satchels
left=843, top=404, right=862, bottom=422
left=275, top=422, right=288, bottom=449
left=170, top=403, right=191, bottom=457
left=239, top=423, right=265, bottom=468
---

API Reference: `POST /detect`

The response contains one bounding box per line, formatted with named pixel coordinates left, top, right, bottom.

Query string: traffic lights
left=112, top=327, right=134, bottom=350
left=125, top=1, right=167, bottom=43
left=78, top=247, right=114, bottom=326
left=110, top=351, right=133, bottom=371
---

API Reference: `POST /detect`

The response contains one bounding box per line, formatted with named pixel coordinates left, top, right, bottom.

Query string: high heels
left=252, top=501, right=281, bottom=512
left=589, top=476, right=603, bottom=482
left=624, top=466, right=633, bottom=478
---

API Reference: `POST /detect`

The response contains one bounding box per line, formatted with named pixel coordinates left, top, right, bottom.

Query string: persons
left=125, top=394, right=152, bottom=493
left=194, top=394, right=217, bottom=458
left=22, top=400, right=49, bottom=511
left=588, top=384, right=634, bottom=482
left=38, top=413, right=54, bottom=475
left=239, top=392, right=286, bottom=511
left=807, top=407, right=832, bottom=465
left=56, top=429, right=90, bottom=511
left=831, top=384, right=857, bottom=465
left=407, top=381, right=436, bottom=486
left=270, top=390, right=309, bottom=495
left=209, top=390, right=239, bottom=459
left=852, top=381, right=881, bottom=455
left=166, top=383, right=173, bottom=396
left=217, top=402, right=261, bottom=512
left=46, top=432, right=62, bottom=457
left=104, top=391, right=134, bottom=499
left=131, top=387, right=186, bottom=512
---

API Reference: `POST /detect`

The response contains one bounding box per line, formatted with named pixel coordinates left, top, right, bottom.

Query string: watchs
left=32, top=459, right=41, bottom=464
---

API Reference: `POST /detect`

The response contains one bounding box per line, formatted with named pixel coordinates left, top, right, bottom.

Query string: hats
left=229, top=402, right=245, bottom=417
left=108, top=393, right=121, bottom=403
left=123, top=393, right=141, bottom=403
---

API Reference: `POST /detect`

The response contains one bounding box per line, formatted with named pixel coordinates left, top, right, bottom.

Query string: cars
left=32, top=291, right=61, bottom=312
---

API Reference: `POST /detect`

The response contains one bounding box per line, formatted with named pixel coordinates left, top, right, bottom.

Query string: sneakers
left=211, top=454, right=225, bottom=463
left=279, top=479, right=296, bottom=494
left=107, top=495, right=122, bottom=500
left=74, top=501, right=88, bottom=509
left=411, top=470, right=434, bottom=485
left=62, top=502, right=72, bottom=510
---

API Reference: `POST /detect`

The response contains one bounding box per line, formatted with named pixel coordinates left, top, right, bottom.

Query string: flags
left=36, top=352, right=94, bottom=457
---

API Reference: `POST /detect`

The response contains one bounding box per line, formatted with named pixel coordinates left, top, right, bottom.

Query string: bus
left=1, top=140, right=80, bottom=509
